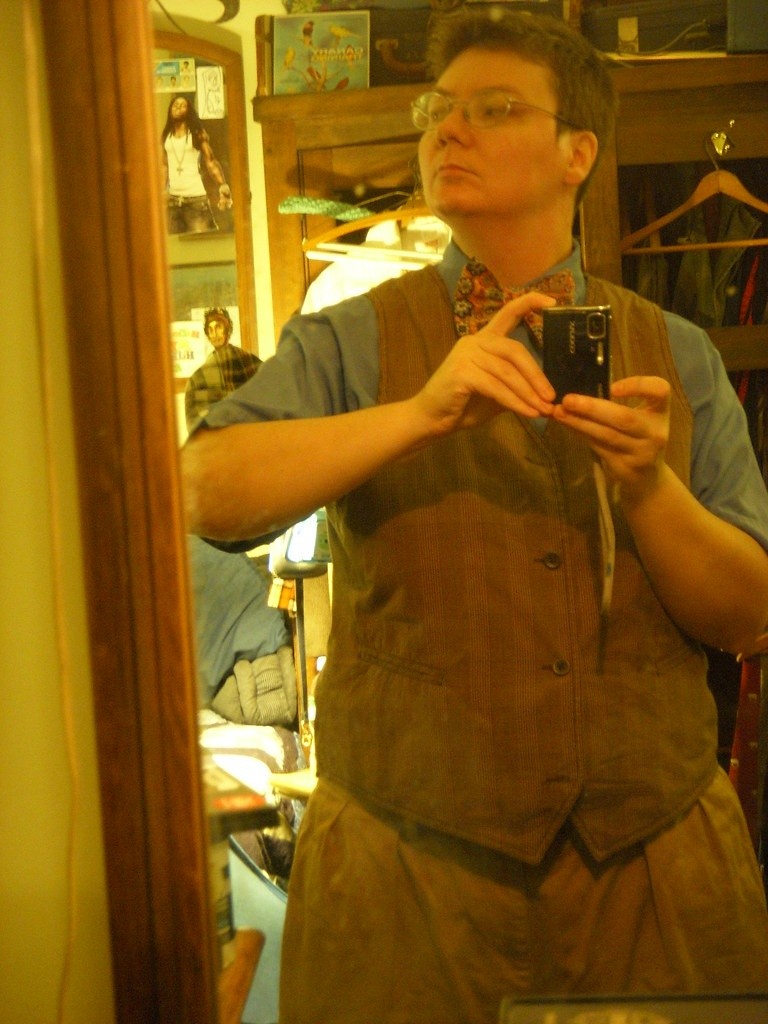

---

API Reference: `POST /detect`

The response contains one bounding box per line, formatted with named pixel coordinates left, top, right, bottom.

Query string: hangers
left=617, top=126, right=768, bottom=255
left=301, top=152, right=451, bottom=271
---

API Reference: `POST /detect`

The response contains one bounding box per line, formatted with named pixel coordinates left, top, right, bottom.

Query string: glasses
left=410, top=91, right=584, bottom=131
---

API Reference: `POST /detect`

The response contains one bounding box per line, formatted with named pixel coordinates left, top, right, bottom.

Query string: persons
left=166, top=77, right=178, bottom=88
left=163, top=96, right=232, bottom=232
left=182, top=62, right=191, bottom=73
left=180, top=12, right=768, bottom=1024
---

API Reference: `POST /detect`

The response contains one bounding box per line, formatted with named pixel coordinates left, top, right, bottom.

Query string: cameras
left=543, top=303, right=611, bottom=402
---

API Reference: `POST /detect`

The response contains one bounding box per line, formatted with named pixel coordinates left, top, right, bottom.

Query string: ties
left=728, top=656, right=758, bottom=857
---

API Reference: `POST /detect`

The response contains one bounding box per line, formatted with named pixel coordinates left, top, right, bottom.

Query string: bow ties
left=453, top=256, right=577, bottom=353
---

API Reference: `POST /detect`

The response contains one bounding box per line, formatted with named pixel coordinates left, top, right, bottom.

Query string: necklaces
left=171, top=132, right=191, bottom=176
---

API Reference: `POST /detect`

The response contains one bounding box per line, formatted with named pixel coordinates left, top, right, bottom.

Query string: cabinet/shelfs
left=250, top=51, right=768, bottom=787
